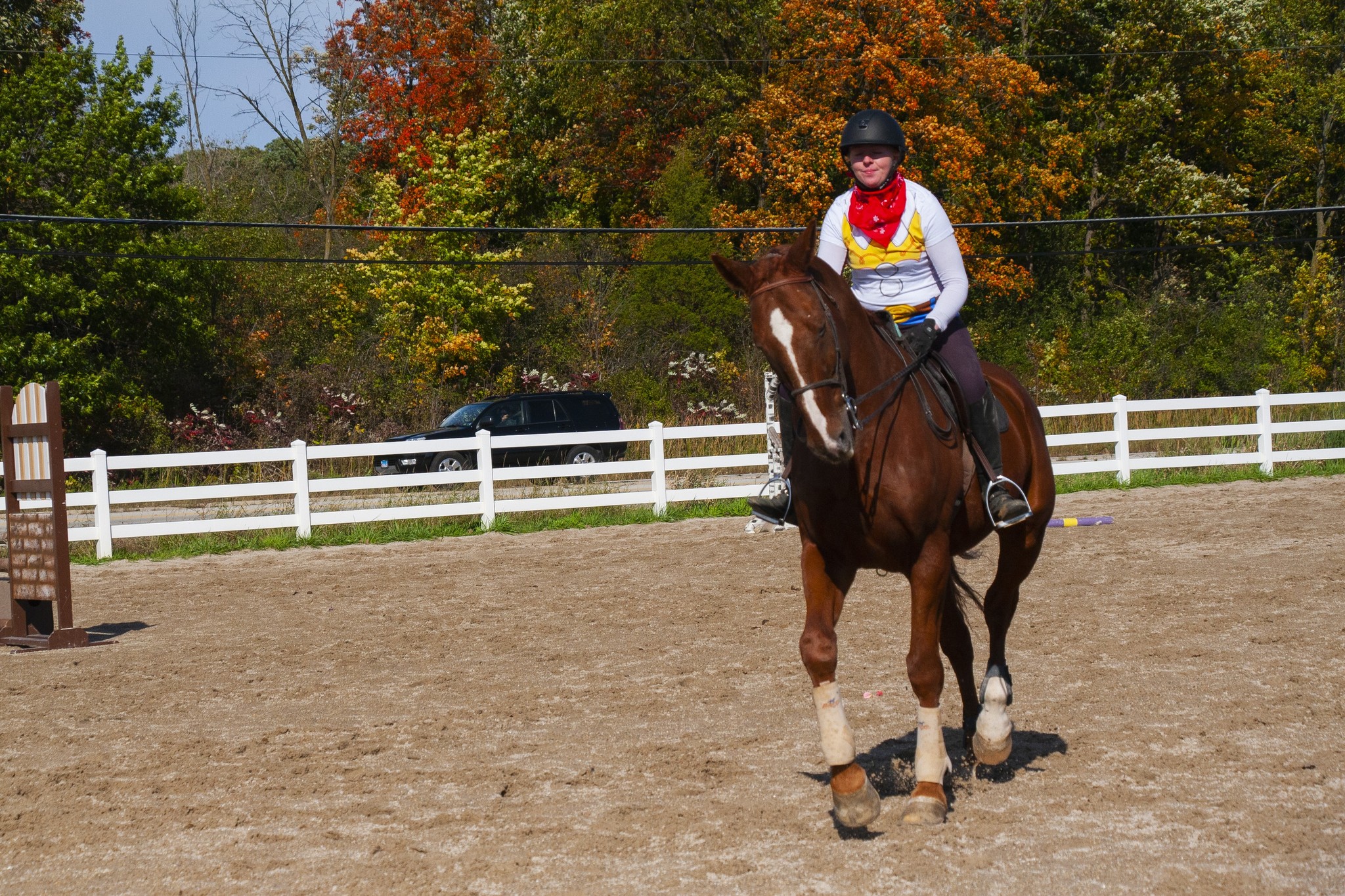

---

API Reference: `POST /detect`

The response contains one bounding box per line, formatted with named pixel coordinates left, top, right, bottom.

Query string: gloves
left=898, top=319, right=942, bottom=359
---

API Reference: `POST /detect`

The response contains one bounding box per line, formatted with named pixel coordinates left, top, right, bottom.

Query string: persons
left=742, top=104, right=1034, bottom=526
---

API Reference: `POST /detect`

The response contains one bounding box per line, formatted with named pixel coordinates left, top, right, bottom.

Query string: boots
left=961, top=381, right=1029, bottom=524
left=748, top=394, right=797, bottom=527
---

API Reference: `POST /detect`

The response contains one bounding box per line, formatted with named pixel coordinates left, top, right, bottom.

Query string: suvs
left=373, top=389, right=627, bottom=493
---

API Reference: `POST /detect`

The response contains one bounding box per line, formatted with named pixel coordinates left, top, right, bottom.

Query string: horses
left=712, top=220, right=1055, bottom=827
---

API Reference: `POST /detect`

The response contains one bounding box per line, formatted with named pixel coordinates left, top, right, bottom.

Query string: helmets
left=839, top=109, right=905, bottom=156
